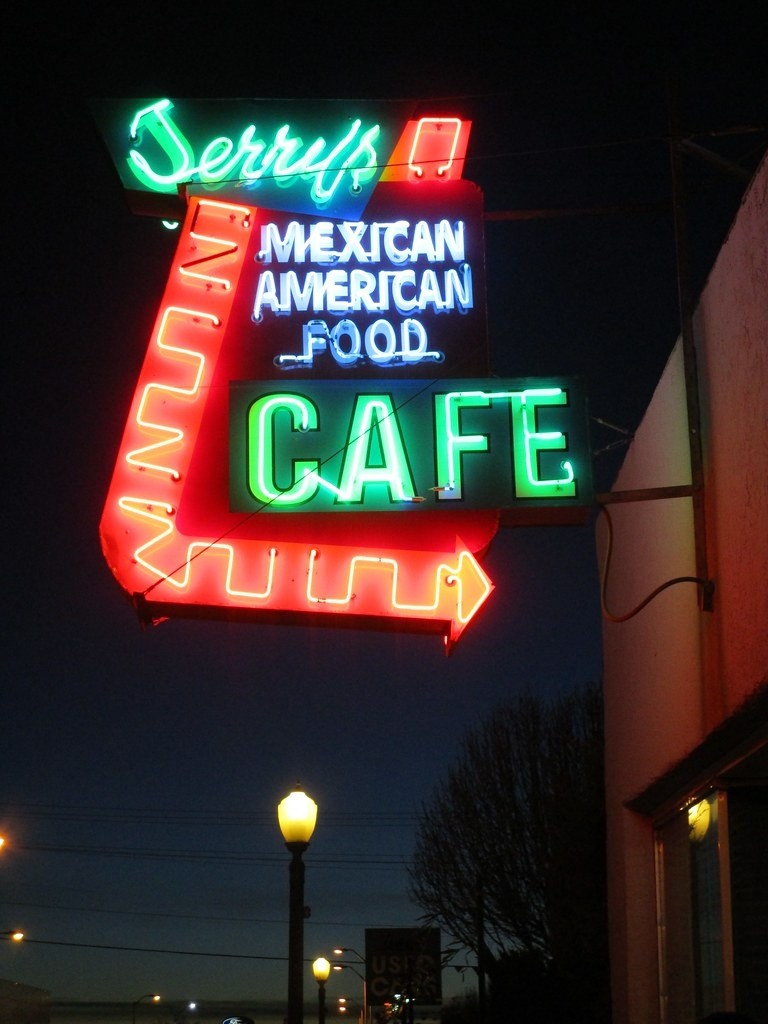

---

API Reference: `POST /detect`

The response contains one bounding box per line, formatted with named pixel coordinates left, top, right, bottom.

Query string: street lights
left=132, top=994, right=161, bottom=1024
left=312, top=952, right=331, bottom=1024
left=277, top=778, right=319, bottom=1024
